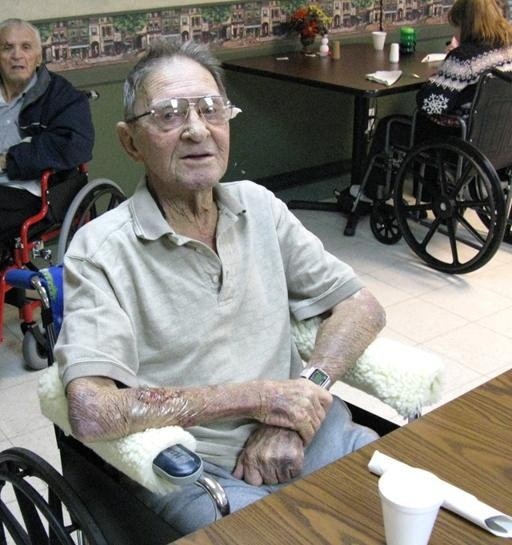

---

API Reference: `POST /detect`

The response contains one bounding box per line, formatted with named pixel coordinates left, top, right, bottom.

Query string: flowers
left=279, top=3, right=334, bottom=40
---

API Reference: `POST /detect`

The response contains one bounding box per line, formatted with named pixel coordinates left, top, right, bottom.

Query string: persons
left=0, top=16, right=97, bottom=273
left=349, top=0, right=512, bottom=203
left=50, top=42, right=387, bottom=533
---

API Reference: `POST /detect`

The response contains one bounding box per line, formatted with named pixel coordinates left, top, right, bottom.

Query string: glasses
left=127, top=95, right=232, bottom=126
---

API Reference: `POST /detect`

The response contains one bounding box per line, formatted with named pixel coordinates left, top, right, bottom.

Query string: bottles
left=389, top=42, right=400, bottom=64
left=331, top=40, right=341, bottom=61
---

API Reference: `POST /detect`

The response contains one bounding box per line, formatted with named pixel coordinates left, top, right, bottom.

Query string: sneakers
left=349, top=184, right=373, bottom=204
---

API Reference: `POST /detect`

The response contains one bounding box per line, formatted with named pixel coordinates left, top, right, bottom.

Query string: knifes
left=485, top=516, right=506, bottom=534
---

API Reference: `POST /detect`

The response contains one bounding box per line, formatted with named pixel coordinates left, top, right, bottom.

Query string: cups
left=399, top=26, right=416, bottom=56
left=371, top=31, right=387, bottom=51
left=378, top=469, right=444, bottom=545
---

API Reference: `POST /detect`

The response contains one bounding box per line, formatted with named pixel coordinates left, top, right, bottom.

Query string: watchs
left=298, top=365, right=333, bottom=393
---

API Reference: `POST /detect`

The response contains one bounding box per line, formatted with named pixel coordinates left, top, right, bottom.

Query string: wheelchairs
left=0, top=261, right=446, bottom=545
left=333, top=67, right=512, bottom=275
left=1, top=89, right=128, bottom=371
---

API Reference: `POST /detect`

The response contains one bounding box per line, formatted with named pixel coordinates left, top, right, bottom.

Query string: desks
left=164, top=369, right=512, bottom=545
left=221, top=37, right=458, bottom=236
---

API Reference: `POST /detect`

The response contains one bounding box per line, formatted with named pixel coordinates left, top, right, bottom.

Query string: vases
left=300, top=34, right=316, bottom=54
